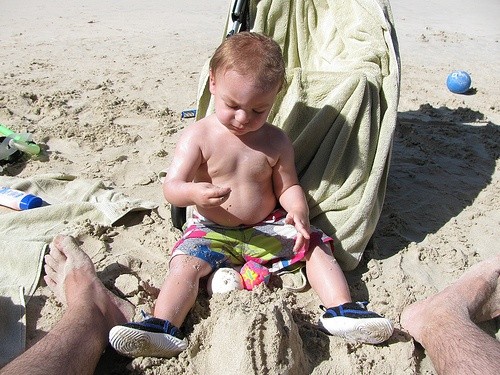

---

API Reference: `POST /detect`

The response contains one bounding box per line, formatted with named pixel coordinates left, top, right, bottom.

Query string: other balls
left=447, top=70, right=471, bottom=93
left=241, top=261, right=270, bottom=291
left=207, top=268, right=243, bottom=295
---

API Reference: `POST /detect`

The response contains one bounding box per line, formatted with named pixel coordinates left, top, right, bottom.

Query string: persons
left=107, top=31, right=395, bottom=358
left=1, top=233, right=500, bottom=375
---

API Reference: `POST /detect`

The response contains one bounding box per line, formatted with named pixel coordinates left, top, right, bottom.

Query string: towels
left=0, top=169, right=158, bottom=372
left=196, top=0, right=402, bottom=272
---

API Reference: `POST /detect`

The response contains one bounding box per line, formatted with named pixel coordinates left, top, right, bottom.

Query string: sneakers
left=316, top=301, right=393, bottom=344
left=107, top=317, right=189, bottom=359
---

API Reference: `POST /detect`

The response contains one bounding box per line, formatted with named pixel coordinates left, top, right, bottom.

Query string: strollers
left=170, top=1, right=402, bottom=245
